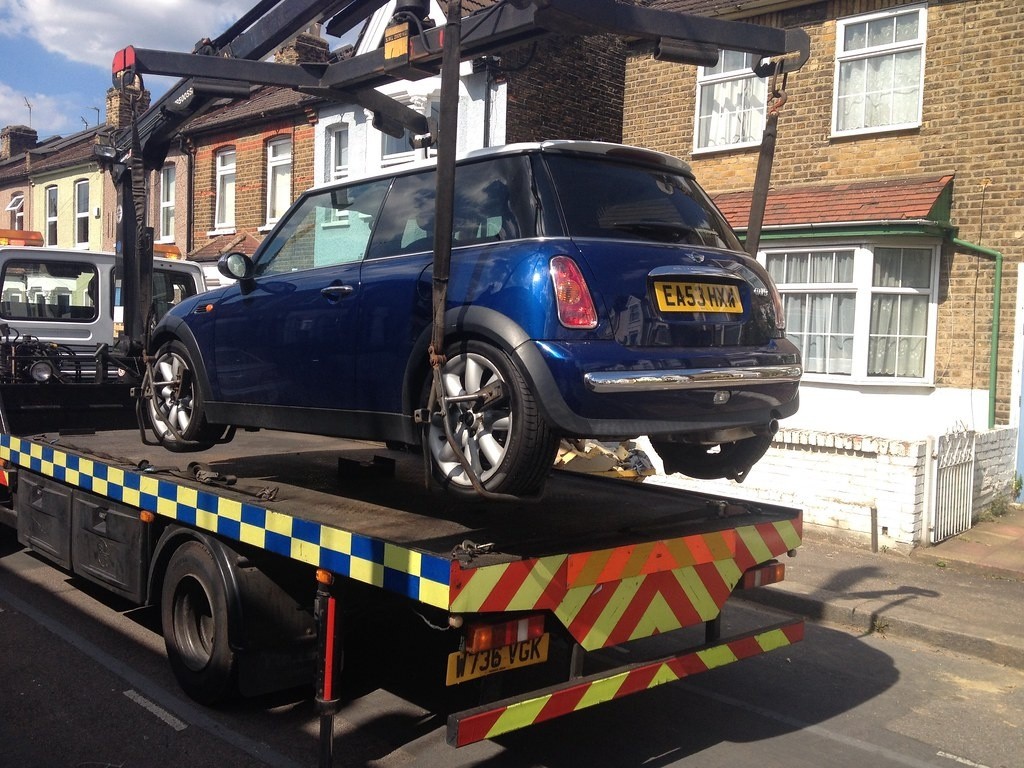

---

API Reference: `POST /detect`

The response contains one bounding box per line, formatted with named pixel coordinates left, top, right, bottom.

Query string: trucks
left=0, top=226, right=811, bottom=768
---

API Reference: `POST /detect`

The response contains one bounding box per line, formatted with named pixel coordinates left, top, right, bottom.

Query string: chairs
left=401, top=194, right=467, bottom=253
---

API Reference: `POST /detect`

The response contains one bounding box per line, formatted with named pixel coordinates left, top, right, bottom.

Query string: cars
left=139, top=138, right=803, bottom=503
